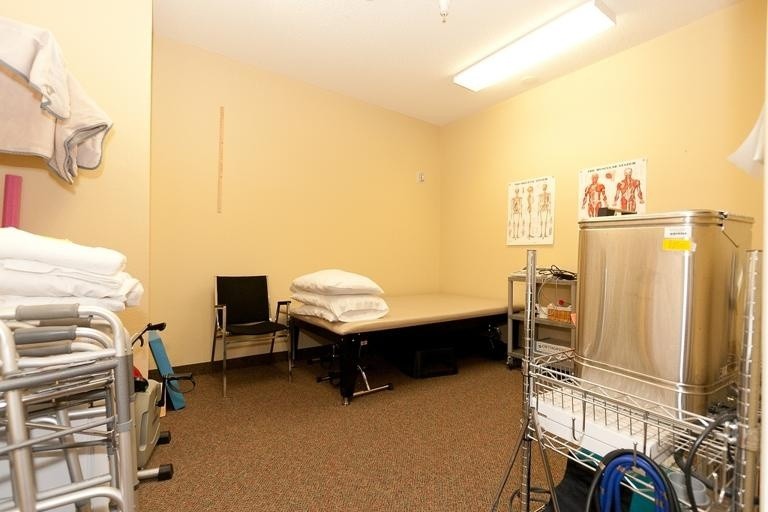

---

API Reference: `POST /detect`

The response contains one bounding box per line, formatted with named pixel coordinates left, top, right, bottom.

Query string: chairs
left=209, top=275, right=292, bottom=398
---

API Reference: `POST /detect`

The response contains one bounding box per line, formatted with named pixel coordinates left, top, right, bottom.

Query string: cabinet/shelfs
left=506, top=273, right=577, bottom=373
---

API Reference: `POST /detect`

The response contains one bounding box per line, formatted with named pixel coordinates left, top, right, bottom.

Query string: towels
left=0, top=17, right=113, bottom=184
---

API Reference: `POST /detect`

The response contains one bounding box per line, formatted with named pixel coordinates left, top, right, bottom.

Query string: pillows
left=291, top=292, right=389, bottom=322
left=292, top=304, right=339, bottom=322
left=291, top=269, right=384, bottom=296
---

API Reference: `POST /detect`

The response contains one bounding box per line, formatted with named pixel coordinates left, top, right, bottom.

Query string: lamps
left=451, top=0, right=616, bottom=94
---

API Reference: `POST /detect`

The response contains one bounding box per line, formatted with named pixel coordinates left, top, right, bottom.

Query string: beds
left=292, top=291, right=524, bottom=405
